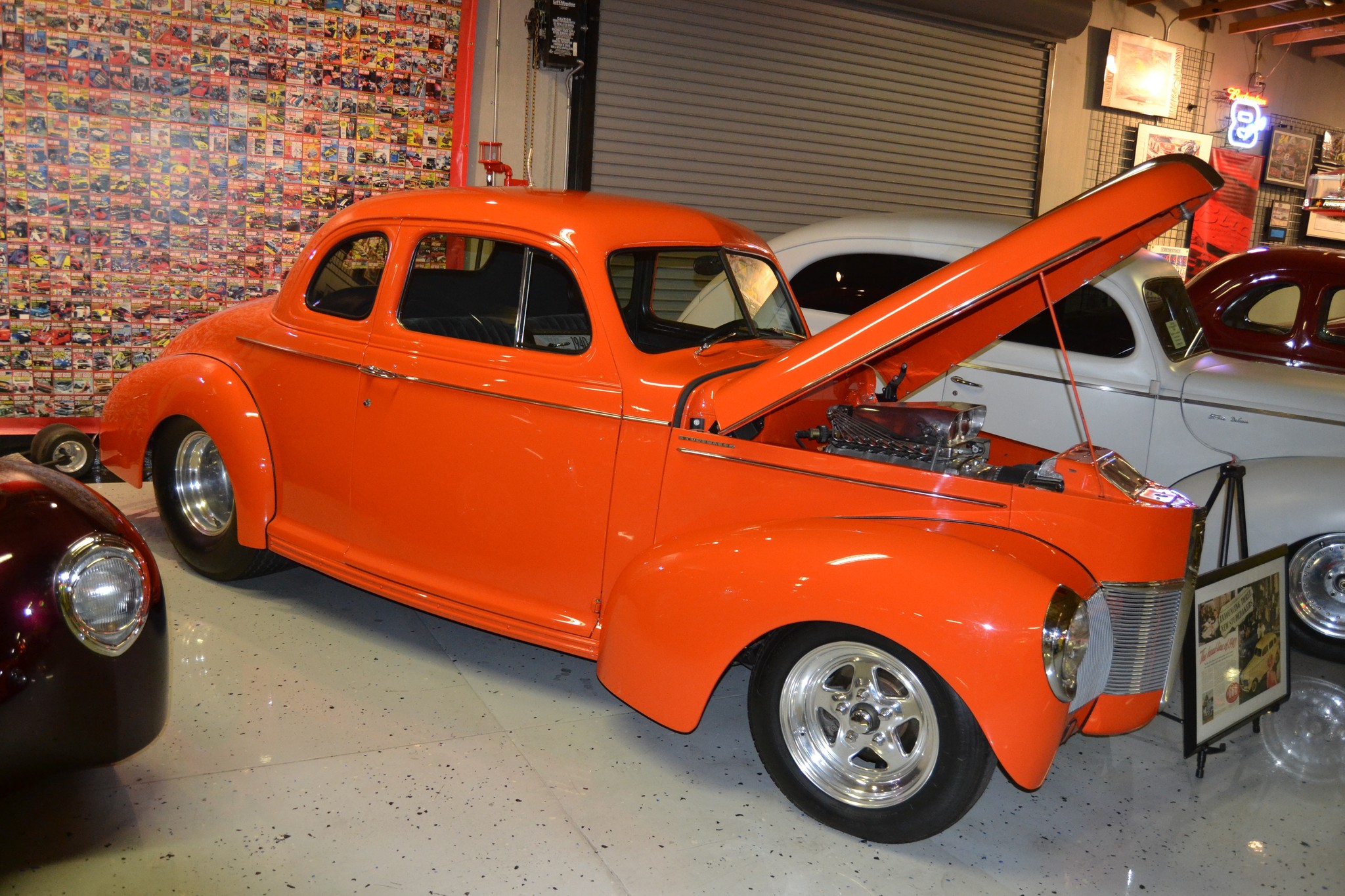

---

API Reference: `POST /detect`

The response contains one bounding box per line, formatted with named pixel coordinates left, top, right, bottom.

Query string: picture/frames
left=1269, top=200, right=1292, bottom=229
left=1264, top=126, right=1317, bottom=191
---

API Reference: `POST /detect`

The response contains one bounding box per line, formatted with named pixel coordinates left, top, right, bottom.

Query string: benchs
left=312, top=284, right=589, bottom=357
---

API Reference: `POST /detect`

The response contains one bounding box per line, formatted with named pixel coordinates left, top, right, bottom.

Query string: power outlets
left=1254, top=75, right=1264, bottom=87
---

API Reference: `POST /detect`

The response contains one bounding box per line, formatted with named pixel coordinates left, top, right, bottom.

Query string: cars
left=1183, top=244, right=1345, bottom=377
left=116, top=148, right=1228, bottom=833
left=679, top=213, right=1345, bottom=666
left=0, top=450, right=170, bottom=772
